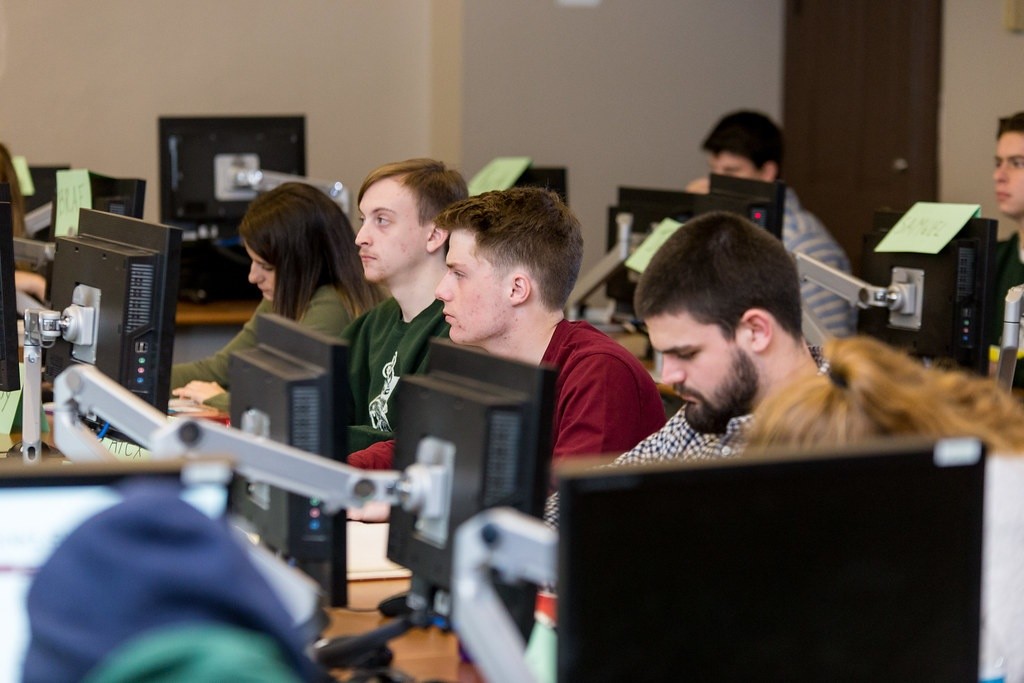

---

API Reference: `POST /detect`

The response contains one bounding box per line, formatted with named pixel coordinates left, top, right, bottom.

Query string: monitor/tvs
left=0, top=114, right=1000, bottom=683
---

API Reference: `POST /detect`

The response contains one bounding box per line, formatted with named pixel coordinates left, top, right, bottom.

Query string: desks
left=169, top=297, right=261, bottom=368
left=314, top=578, right=460, bottom=683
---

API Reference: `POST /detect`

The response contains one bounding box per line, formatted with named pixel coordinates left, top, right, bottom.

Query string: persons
left=23, top=493, right=323, bottom=683
left=544, top=212, right=831, bottom=594
left=991, top=111, right=1024, bottom=349
left=743, top=337, right=1023, bottom=683
left=346, top=189, right=668, bottom=521
left=686, top=108, right=850, bottom=342
left=337, top=158, right=468, bottom=454
left=170, top=181, right=386, bottom=413
